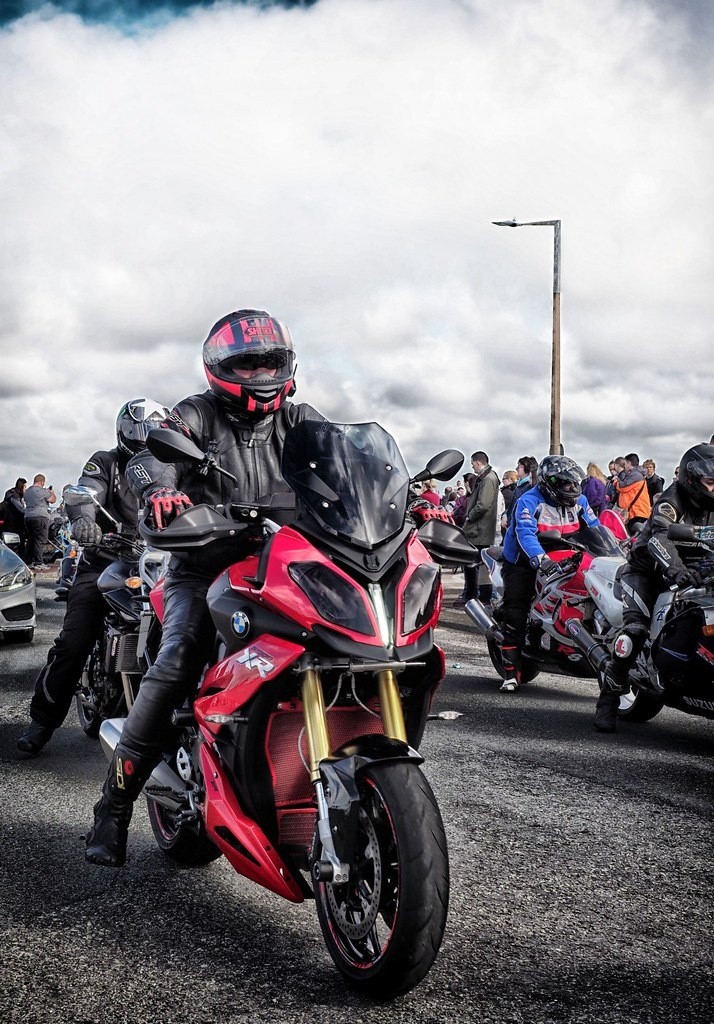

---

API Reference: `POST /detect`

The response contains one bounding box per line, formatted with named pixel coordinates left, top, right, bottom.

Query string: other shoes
left=30, top=562, right=51, bottom=570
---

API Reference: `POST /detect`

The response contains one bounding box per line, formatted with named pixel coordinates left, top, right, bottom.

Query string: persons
left=453, top=452, right=500, bottom=607
left=17, top=399, right=172, bottom=754
left=85, top=309, right=456, bottom=867
left=3, top=477, right=26, bottom=533
left=501, top=456, right=603, bottom=692
left=596, top=434, right=714, bottom=731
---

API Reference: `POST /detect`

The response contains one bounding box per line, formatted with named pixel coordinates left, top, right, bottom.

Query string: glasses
left=503, top=478, right=508, bottom=480
left=230, top=354, right=288, bottom=371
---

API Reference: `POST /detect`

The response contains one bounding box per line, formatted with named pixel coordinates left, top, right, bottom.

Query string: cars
left=0, top=529, right=38, bottom=644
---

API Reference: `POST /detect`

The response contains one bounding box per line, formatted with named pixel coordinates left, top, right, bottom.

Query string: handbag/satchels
left=611, top=503, right=630, bottom=524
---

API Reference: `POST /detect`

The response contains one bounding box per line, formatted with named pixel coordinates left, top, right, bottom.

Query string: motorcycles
left=464, top=521, right=714, bottom=722
left=64, top=483, right=168, bottom=738
left=42, top=503, right=88, bottom=599
left=99, top=420, right=481, bottom=998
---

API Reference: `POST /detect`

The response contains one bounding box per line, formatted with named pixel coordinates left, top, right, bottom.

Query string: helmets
left=679, top=444, right=714, bottom=512
left=203, top=310, right=296, bottom=413
left=537, top=455, right=587, bottom=507
left=116, top=399, right=170, bottom=458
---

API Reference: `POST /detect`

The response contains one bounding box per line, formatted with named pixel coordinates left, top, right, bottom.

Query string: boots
left=594, top=665, right=629, bottom=730
left=17, top=720, right=52, bottom=753
left=453, top=585, right=478, bottom=606
left=499, top=647, right=521, bottom=692
left=86, top=721, right=165, bottom=867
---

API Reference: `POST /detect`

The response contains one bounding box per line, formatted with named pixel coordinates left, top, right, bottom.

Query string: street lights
left=495, top=219, right=561, bottom=455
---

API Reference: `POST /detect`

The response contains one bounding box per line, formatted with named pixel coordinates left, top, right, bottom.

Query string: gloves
left=408, top=499, right=455, bottom=525
left=675, top=569, right=704, bottom=589
left=71, top=518, right=101, bottom=544
left=537, top=554, right=563, bottom=577
left=142, top=487, right=194, bottom=529
